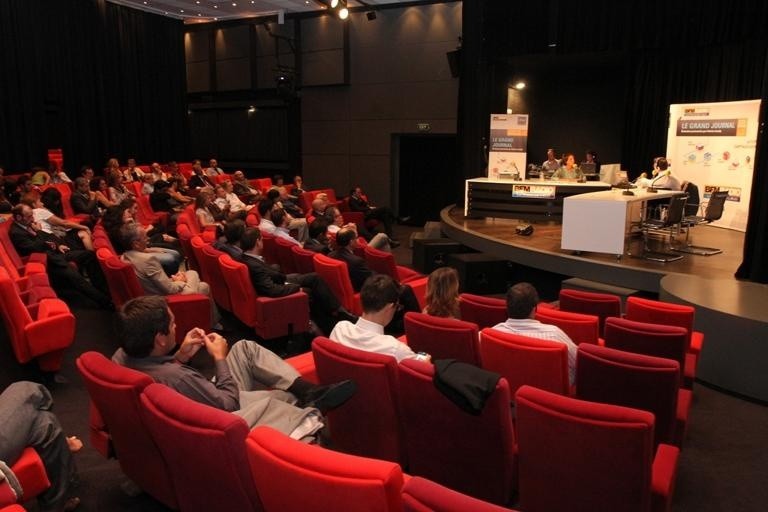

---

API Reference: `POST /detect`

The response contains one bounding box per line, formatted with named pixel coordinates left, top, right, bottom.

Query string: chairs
left=0, top=164, right=431, bottom=512
left=674, top=189, right=730, bottom=255
left=396, top=288, right=702, bottom=512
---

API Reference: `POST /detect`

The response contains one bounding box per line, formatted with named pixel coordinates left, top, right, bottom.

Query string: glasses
left=395, top=303, right=405, bottom=312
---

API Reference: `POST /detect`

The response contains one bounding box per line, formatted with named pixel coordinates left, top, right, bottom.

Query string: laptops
left=582, top=164, right=596, bottom=174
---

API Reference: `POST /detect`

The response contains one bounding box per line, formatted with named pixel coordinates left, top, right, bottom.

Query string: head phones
left=592, top=151, right=596, bottom=158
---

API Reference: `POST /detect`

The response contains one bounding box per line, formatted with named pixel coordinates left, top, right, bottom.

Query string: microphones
left=627, top=171, right=646, bottom=188
left=572, top=163, right=585, bottom=183
left=510, top=161, right=522, bottom=181
left=651, top=170, right=657, bottom=179
left=647, top=170, right=671, bottom=193
left=623, top=179, right=637, bottom=195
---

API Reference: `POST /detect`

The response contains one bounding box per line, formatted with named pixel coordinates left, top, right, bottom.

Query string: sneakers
left=390, top=241, right=400, bottom=248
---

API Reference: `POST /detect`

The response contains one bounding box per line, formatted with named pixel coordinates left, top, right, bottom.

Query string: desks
left=560, top=186, right=690, bottom=263
left=464, top=177, right=611, bottom=221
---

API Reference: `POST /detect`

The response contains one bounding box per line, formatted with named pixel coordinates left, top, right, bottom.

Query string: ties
left=201, top=177, right=209, bottom=186
left=27, top=227, right=57, bottom=251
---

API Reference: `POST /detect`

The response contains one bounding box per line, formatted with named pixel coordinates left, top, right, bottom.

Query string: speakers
left=446, top=50, right=464, bottom=78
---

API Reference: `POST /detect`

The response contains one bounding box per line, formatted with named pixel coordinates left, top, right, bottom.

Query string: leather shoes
left=64, top=496, right=81, bottom=512
left=301, top=379, right=356, bottom=416
left=210, top=321, right=233, bottom=333
left=332, top=310, right=359, bottom=324
left=65, top=436, right=84, bottom=452
left=396, top=215, right=410, bottom=224
